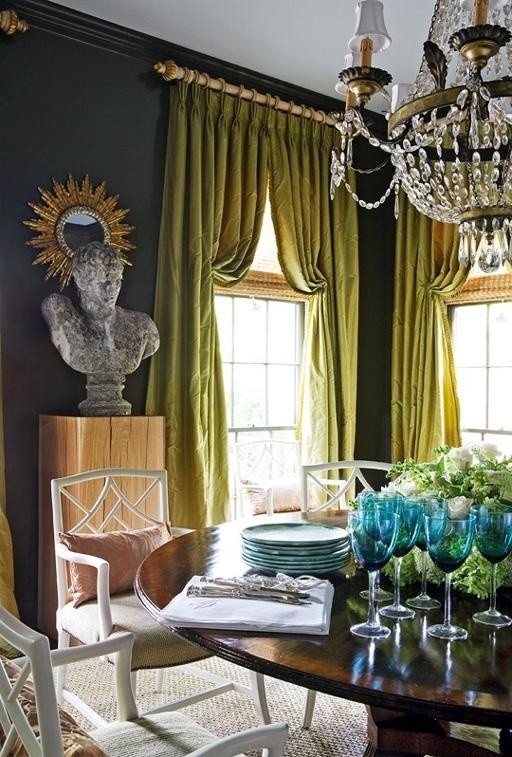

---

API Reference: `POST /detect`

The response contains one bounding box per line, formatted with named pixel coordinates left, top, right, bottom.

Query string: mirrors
left=20, top=172, right=137, bottom=296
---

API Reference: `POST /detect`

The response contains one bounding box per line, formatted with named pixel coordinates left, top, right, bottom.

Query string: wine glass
left=347, top=491, right=512, bottom=642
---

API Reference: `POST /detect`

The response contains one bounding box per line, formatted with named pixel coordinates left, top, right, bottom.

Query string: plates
left=239, top=522, right=351, bottom=576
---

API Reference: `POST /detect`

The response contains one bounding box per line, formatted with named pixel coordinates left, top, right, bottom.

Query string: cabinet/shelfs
left=37, top=415, right=166, bottom=640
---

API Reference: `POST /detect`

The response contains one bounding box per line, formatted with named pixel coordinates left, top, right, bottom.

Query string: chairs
left=301, top=460, right=400, bottom=729
left=50, top=467, right=271, bottom=729
left=1, top=605, right=288, bottom=757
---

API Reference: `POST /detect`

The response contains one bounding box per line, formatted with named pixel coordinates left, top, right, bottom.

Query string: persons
left=40, top=240, right=161, bottom=376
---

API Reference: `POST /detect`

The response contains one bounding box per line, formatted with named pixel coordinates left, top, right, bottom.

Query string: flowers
left=349, top=442, right=512, bottom=602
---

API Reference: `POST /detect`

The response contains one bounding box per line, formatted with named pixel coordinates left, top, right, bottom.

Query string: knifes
left=186, top=575, right=310, bottom=607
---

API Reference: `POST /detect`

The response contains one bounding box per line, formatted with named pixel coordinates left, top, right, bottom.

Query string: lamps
left=330, top=0, right=511, bottom=274
